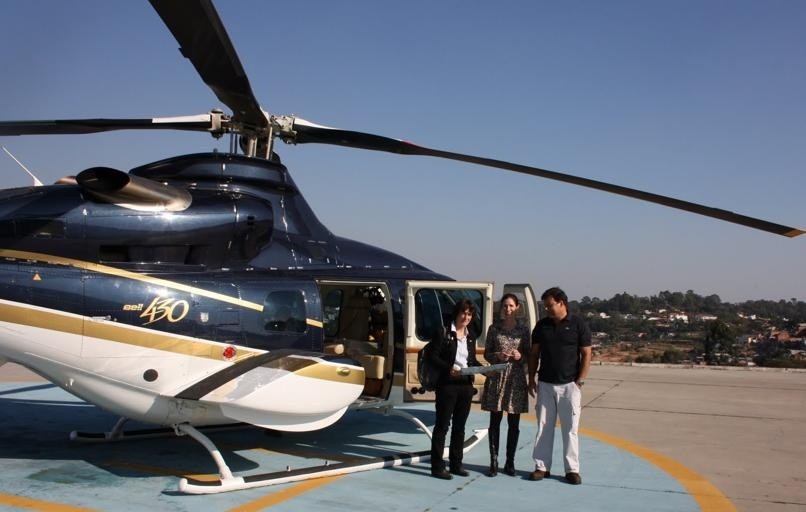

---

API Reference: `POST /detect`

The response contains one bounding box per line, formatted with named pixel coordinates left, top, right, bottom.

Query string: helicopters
left=0, top=0, right=804, bottom=496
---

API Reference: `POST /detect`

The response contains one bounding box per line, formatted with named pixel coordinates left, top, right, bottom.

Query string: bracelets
left=578, top=381, right=584, bottom=386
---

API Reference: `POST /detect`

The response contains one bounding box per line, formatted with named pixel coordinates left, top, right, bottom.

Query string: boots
left=488, top=428, right=499, bottom=477
left=504, top=429, right=519, bottom=475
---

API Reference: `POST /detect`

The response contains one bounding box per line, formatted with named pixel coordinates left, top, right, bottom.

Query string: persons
left=427, top=297, right=495, bottom=479
left=481, top=294, right=530, bottom=477
left=528, top=287, right=592, bottom=484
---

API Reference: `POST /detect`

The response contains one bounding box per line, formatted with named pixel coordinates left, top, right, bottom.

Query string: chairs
left=360, top=355, right=385, bottom=396
left=324, top=343, right=345, bottom=357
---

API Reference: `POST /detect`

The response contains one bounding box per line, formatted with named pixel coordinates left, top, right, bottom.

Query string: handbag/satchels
left=417, top=327, right=450, bottom=391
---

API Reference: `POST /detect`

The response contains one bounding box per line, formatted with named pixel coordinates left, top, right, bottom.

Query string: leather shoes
left=450, top=464, right=468, bottom=476
left=432, top=467, right=451, bottom=479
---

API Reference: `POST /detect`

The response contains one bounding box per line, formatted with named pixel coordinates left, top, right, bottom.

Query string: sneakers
left=567, top=472, right=581, bottom=484
left=530, top=469, right=550, bottom=480
